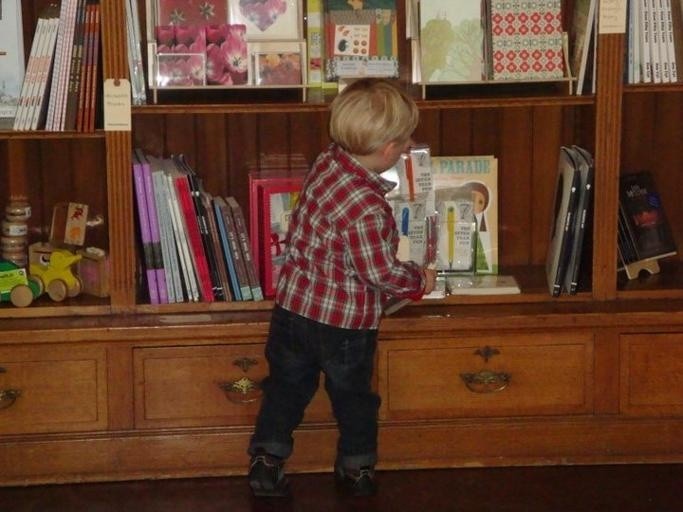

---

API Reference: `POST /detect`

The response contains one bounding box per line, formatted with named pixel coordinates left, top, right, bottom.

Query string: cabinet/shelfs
left=0, top=0, right=683, bottom=487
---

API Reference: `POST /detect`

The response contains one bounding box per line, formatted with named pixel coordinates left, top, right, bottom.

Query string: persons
left=246, top=77, right=437, bottom=509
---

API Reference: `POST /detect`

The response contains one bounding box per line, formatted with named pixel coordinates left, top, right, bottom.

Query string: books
left=1, top=0, right=102, bottom=133
left=547, top=145, right=593, bottom=296
left=132, top=149, right=307, bottom=306
left=122, top=1, right=594, bottom=106
left=628, top=0, right=678, bottom=84
left=376, top=147, right=521, bottom=298
left=618, top=170, right=678, bottom=269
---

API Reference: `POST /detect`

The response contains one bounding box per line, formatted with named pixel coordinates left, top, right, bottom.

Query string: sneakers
left=248, top=452, right=288, bottom=497
left=335, top=464, right=377, bottom=496
